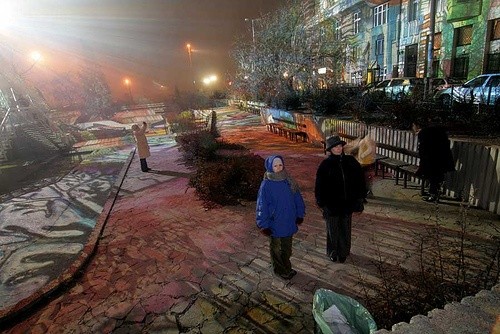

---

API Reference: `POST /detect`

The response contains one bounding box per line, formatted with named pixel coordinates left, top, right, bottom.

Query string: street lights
left=244, top=18, right=260, bottom=71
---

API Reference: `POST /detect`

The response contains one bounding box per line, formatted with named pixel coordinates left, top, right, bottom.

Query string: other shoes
left=288, top=269, right=297, bottom=275
left=426, top=196, right=436, bottom=202
left=330, top=256, right=338, bottom=262
left=281, top=272, right=293, bottom=279
left=339, top=256, right=347, bottom=263
left=147, top=168, right=151, bottom=170
left=143, top=169, right=148, bottom=172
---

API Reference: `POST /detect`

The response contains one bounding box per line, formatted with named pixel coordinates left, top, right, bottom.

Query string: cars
left=344, top=76, right=448, bottom=108
left=433, top=73, right=500, bottom=118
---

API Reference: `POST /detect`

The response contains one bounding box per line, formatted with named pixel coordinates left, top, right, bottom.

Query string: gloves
left=297, top=218, right=303, bottom=226
left=263, top=228, right=274, bottom=237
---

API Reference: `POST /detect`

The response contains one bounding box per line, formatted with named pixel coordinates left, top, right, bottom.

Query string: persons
left=412, top=118, right=445, bottom=202
left=358, top=129, right=377, bottom=196
left=256, top=155, right=305, bottom=279
left=314, top=136, right=364, bottom=263
left=131, top=122, right=152, bottom=172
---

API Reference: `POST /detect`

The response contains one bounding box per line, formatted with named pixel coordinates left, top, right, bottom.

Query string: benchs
left=320, top=141, right=425, bottom=194
left=266, top=123, right=303, bottom=143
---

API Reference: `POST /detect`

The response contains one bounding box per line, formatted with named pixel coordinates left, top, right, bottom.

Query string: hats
left=325, top=134, right=346, bottom=152
